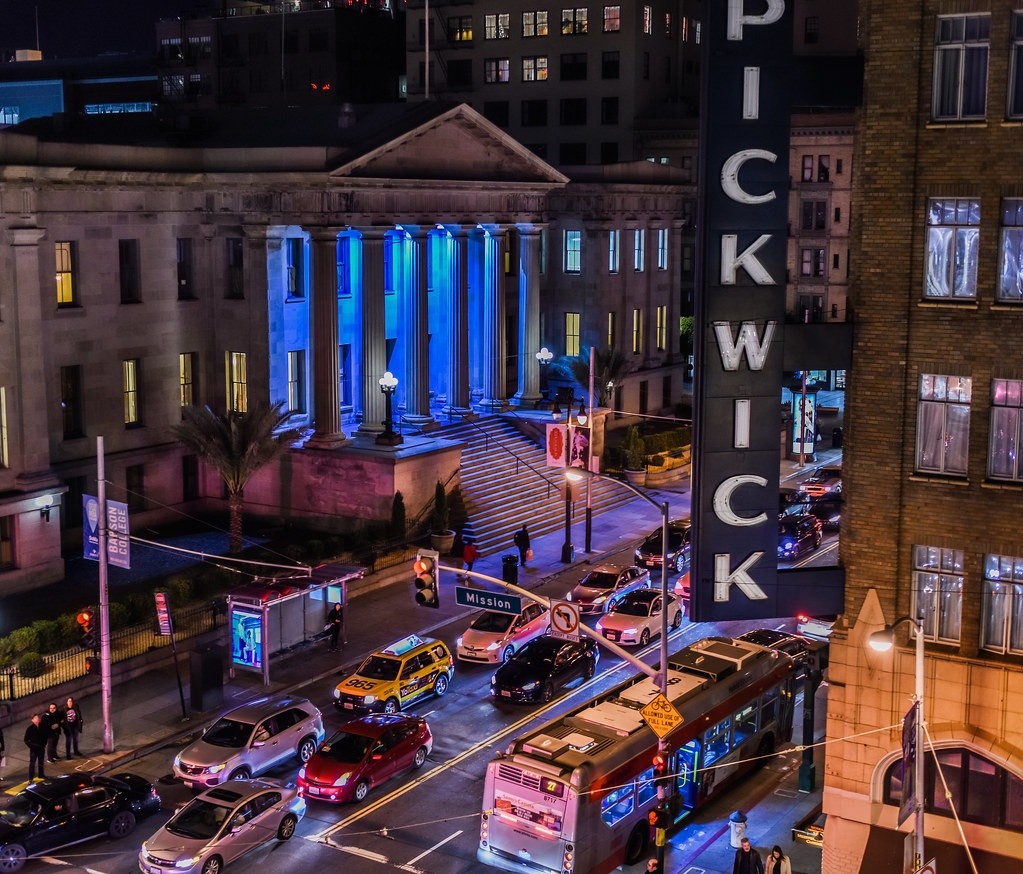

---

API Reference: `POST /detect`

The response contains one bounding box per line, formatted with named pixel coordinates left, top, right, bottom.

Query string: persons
left=796, top=397, right=815, bottom=443
left=231, top=812, right=245, bottom=827
left=574, top=429, right=587, bottom=459
left=41, top=703, right=62, bottom=765
left=45, top=797, right=70, bottom=822
left=784, top=400, right=791, bottom=404
left=364, top=738, right=387, bottom=754
left=252, top=723, right=270, bottom=744
left=644, top=858, right=664, bottom=874
left=206, top=600, right=218, bottom=630
left=462, top=539, right=479, bottom=580
left=815, top=420, right=822, bottom=445
left=816, top=403, right=822, bottom=407
left=326, top=602, right=343, bottom=653
left=24, top=714, right=48, bottom=785
left=60, top=697, right=83, bottom=760
left=514, top=524, right=534, bottom=567
left=733, top=838, right=763, bottom=874
left=764, top=845, right=792, bottom=874
left=0, top=723, right=6, bottom=780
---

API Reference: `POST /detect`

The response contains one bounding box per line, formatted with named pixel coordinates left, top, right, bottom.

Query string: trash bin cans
left=502, top=554, right=518, bottom=584
left=832, top=427, right=843, bottom=448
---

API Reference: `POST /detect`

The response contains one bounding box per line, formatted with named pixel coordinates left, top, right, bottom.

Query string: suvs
left=738, top=626, right=824, bottom=671
left=776, top=513, right=823, bottom=560
left=808, top=491, right=842, bottom=532
left=632, top=518, right=692, bottom=574
left=173, top=694, right=325, bottom=794
left=798, top=465, right=842, bottom=497
left=334, top=633, right=454, bottom=714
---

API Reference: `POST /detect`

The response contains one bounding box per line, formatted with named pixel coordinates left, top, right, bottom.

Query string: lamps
left=535, top=348, right=554, bottom=405
left=61, top=399, right=70, bottom=409
left=375, top=372, right=403, bottom=446
left=40, top=495, right=53, bottom=522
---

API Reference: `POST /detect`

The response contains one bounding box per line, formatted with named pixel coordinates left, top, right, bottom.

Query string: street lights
left=865, top=616, right=924, bottom=874
left=533, top=348, right=557, bottom=410
left=562, top=467, right=669, bottom=874
left=374, top=371, right=403, bottom=445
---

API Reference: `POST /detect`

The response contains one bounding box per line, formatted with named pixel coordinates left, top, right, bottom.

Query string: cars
left=455, top=594, right=567, bottom=666
left=0, top=769, right=161, bottom=874
left=778, top=487, right=811, bottom=521
left=489, top=631, right=601, bottom=706
left=138, top=777, right=308, bottom=874
left=595, top=587, right=685, bottom=647
left=296, top=712, right=434, bottom=805
left=564, top=562, right=652, bottom=614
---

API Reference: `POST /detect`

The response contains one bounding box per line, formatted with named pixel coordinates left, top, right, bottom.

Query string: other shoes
left=53, top=755, right=60, bottom=761
left=47, top=759, right=55, bottom=763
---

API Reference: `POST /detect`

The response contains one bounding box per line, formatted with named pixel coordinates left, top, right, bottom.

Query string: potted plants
left=617, top=424, right=648, bottom=487
left=430, top=485, right=457, bottom=553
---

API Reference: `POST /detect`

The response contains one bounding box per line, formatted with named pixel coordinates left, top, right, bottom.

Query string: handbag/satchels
left=526, top=548, right=536, bottom=562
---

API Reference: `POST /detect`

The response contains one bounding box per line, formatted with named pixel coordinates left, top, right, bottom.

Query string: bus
left=477, top=635, right=796, bottom=874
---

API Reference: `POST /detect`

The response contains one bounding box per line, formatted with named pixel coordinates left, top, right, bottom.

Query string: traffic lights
left=84, top=656, right=100, bottom=674
left=412, top=549, right=440, bottom=609
left=76, top=607, right=99, bottom=649
left=652, top=751, right=669, bottom=787
left=647, top=809, right=669, bottom=828
